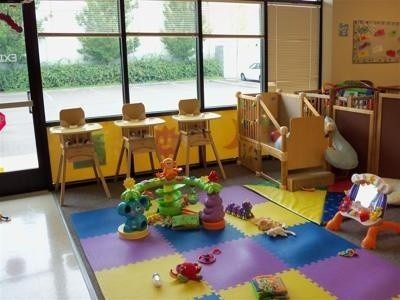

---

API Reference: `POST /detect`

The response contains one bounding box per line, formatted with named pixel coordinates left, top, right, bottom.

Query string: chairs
left=114, top=102, right=168, bottom=183
left=49, top=107, right=112, bottom=206
left=171, top=99, right=227, bottom=180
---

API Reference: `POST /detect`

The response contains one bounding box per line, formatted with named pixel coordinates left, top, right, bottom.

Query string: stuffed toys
left=169, top=261, right=204, bottom=282
left=338, top=249, right=360, bottom=257
left=255, top=216, right=297, bottom=239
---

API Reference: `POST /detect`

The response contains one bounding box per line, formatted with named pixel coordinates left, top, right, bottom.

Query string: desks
left=49, top=123, right=103, bottom=135
left=172, top=112, right=222, bottom=122
left=113, top=117, right=167, bottom=128
